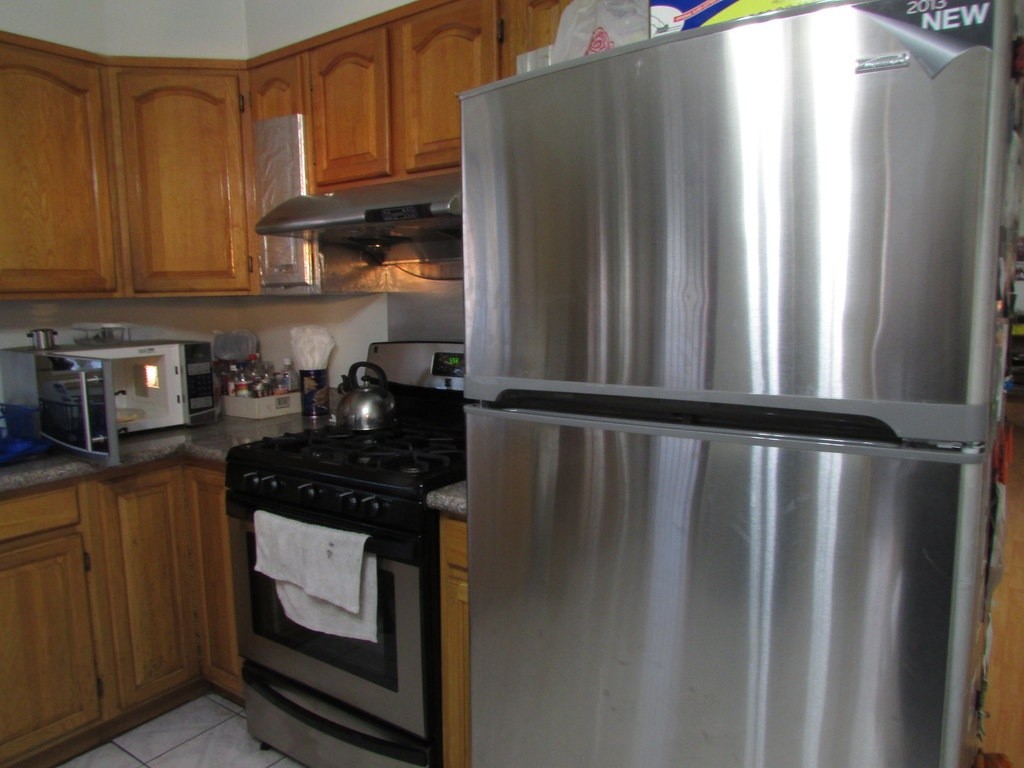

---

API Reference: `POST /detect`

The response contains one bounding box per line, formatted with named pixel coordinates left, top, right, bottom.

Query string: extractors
left=255, top=169, right=461, bottom=248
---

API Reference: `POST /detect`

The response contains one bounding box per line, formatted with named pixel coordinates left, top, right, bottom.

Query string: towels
left=255, top=510, right=376, bottom=643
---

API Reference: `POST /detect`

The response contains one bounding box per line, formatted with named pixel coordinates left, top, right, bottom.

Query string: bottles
left=220, top=353, right=300, bottom=398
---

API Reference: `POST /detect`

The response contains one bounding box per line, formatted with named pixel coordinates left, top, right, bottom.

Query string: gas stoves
left=228, top=342, right=466, bottom=495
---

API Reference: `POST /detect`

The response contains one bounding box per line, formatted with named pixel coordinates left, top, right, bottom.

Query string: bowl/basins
left=70, top=322, right=132, bottom=345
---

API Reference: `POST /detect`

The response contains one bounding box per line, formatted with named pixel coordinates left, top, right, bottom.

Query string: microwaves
left=1, top=339, right=220, bottom=467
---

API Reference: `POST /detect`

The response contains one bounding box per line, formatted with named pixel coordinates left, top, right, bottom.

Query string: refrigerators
left=457, top=4, right=1009, bottom=768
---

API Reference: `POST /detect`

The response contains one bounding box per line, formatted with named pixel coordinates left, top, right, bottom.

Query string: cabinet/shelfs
left=99, top=466, right=202, bottom=710
left=499, top=0, right=578, bottom=81
left=307, top=0, right=499, bottom=194
left=193, top=462, right=470, bottom=768
left=249, top=53, right=324, bottom=297
left=0, top=28, right=259, bottom=301
left=0, top=476, right=105, bottom=768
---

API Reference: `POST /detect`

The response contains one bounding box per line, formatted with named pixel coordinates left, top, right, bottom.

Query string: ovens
left=220, top=489, right=444, bottom=768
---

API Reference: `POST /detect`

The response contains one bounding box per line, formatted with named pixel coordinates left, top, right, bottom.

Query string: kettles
left=336, top=362, right=396, bottom=430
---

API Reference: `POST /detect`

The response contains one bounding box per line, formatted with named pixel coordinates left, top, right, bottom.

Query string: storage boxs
left=223, top=392, right=301, bottom=419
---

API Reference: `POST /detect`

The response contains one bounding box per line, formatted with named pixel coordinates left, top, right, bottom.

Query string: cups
left=26, top=328, right=57, bottom=349
left=299, top=368, right=331, bottom=419
